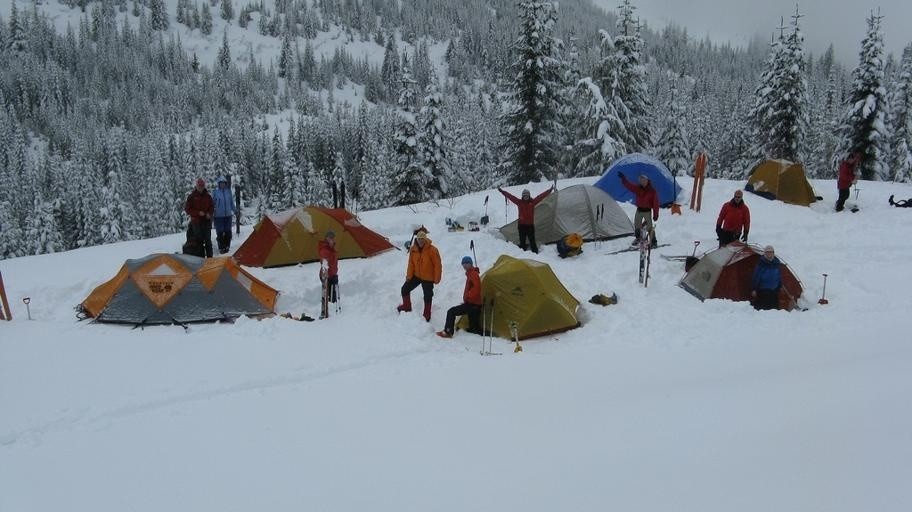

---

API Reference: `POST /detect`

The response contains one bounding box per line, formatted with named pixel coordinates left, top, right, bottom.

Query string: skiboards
left=321, top=261, right=333, bottom=319
left=639, top=224, right=653, bottom=285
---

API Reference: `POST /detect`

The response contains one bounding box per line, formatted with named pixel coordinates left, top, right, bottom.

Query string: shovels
left=817, top=274, right=829, bottom=304
left=511, top=322, right=522, bottom=352
left=481, top=196, right=489, bottom=226
left=685, top=240, right=700, bottom=271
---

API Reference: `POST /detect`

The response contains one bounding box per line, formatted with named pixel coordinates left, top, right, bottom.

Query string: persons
left=715, top=187, right=751, bottom=250
left=184, top=176, right=214, bottom=262
left=212, top=173, right=239, bottom=255
left=394, top=229, right=442, bottom=324
left=315, top=230, right=342, bottom=304
left=615, top=170, right=661, bottom=247
left=498, top=183, right=556, bottom=254
left=833, top=151, right=859, bottom=214
left=749, top=244, right=782, bottom=312
left=433, top=255, right=481, bottom=336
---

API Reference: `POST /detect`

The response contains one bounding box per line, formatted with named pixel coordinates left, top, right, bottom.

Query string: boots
left=396, top=293, right=412, bottom=312
left=217, top=237, right=226, bottom=255
left=224, top=235, right=231, bottom=251
left=424, top=302, right=432, bottom=322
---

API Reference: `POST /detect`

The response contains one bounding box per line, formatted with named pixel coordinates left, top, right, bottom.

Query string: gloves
left=742, top=231, right=749, bottom=242
left=653, top=215, right=658, bottom=220
left=616, top=171, right=623, bottom=178
left=715, top=224, right=722, bottom=235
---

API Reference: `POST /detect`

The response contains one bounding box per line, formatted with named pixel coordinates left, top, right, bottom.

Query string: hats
left=415, top=231, right=426, bottom=239
left=763, top=246, right=774, bottom=253
left=195, top=178, right=204, bottom=186
left=522, top=189, right=530, bottom=196
left=324, top=231, right=335, bottom=238
left=461, top=256, right=473, bottom=264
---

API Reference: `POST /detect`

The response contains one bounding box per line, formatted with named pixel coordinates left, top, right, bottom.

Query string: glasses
left=735, top=196, right=741, bottom=199
left=463, top=261, right=468, bottom=264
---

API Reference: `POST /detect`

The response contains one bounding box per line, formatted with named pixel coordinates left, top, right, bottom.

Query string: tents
left=742, top=156, right=818, bottom=209
left=456, top=253, right=584, bottom=355
left=74, top=250, right=282, bottom=332
left=498, top=184, right=636, bottom=249
left=591, top=150, right=684, bottom=210
left=231, top=205, right=401, bottom=268
left=679, top=238, right=805, bottom=307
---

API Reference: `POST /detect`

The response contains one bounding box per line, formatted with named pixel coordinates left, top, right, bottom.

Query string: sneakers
left=632, top=238, right=639, bottom=245
left=735, top=191, right=743, bottom=195
left=437, top=331, right=452, bottom=338
left=651, top=239, right=658, bottom=248
